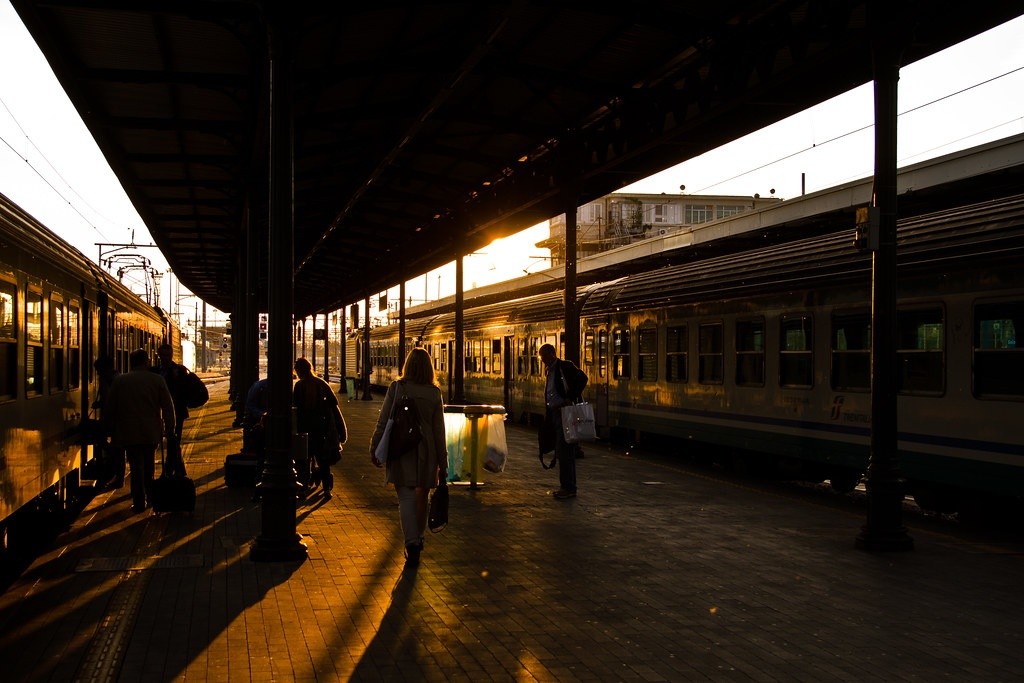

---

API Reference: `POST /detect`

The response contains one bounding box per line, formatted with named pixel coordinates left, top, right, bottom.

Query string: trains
left=0, top=100, right=185, bottom=571
left=342, top=188, right=1023, bottom=530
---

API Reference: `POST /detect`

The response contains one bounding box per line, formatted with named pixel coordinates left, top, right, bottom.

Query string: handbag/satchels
left=175, top=362, right=209, bottom=410
left=562, top=394, right=596, bottom=443
left=391, top=381, right=423, bottom=459
left=536, top=423, right=555, bottom=455
left=428, top=472, right=448, bottom=532
left=374, top=381, right=399, bottom=464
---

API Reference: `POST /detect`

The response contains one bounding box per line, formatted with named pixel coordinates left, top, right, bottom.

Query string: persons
left=113, top=348, right=177, bottom=511
left=92, top=357, right=126, bottom=489
left=369, top=346, right=450, bottom=568
left=540, top=343, right=588, bottom=498
left=292, top=355, right=348, bottom=501
left=150, top=345, right=188, bottom=478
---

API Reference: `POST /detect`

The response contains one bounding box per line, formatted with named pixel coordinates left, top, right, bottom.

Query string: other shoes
left=552, top=490, right=577, bottom=500
left=403, top=540, right=426, bottom=569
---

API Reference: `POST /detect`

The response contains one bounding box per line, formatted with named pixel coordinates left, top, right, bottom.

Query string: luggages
left=148, top=432, right=196, bottom=517
left=260, top=473, right=296, bottom=541
